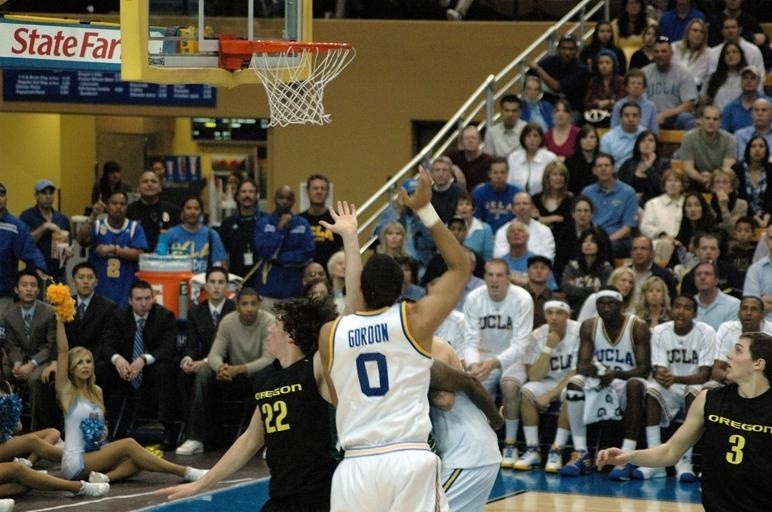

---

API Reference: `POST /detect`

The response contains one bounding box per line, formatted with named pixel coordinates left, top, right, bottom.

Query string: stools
left=12, top=381, right=255, bottom=447
left=507, top=406, right=703, bottom=471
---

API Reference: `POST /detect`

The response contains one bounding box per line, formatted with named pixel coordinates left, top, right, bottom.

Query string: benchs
left=594, top=125, right=714, bottom=205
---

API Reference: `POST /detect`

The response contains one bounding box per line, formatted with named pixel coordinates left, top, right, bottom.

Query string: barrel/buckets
left=134, top=270, right=195, bottom=319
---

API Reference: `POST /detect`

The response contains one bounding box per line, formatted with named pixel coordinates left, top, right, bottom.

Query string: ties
left=129, top=318, right=146, bottom=390
left=211, top=310, right=220, bottom=329
left=23, top=313, right=32, bottom=344
left=78, top=302, right=86, bottom=321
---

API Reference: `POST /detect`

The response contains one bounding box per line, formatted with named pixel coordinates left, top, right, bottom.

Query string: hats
left=655, top=34, right=672, bottom=44
left=35, top=179, right=56, bottom=192
left=400, top=177, right=419, bottom=194
left=739, top=64, right=760, bottom=78
left=560, top=34, right=577, bottom=42
left=527, top=255, right=552, bottom=268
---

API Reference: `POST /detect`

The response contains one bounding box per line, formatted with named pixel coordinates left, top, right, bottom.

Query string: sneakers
left=500, top=440, right=518, bottom=468
left=673, top=454, right=698, bottom=485
left=513, top=445, right=543, bottom=471
left=175, top=438, right=205, bottom=456
left=185, top=466, right=209, bottom=481
left=36, top=469, right=48, bottom=474
left=89, top=470, right=110, bottom=483
left=544, top=444, right=562, bottom=473
left=561, top=449, right=593, bottom=476
left=633, top=461, right=667, bottom=481
left=610, top=460, right=631, bottom=481
left=75, top=479, right=110, bottom=497
left=12, top=457, right=32, bottom=467
left=0, top=498, right=15, bottom=512
